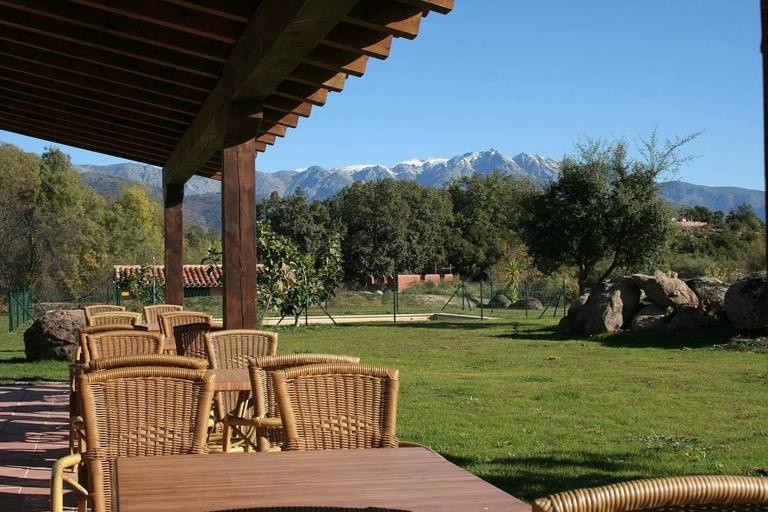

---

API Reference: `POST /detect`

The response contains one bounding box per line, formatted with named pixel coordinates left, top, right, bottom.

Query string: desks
left=112, top=447, right=533, bottom=512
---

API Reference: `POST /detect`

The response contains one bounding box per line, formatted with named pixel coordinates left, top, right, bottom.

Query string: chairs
left=52, top=355, right=211, bottom=511
left=531, top=469, right=767, bottom=512
left=68, top=303, right=277, bottom=444
left=222, top=354, right=361, bottom=452
left=276, top=364, right=397, bottom=451
left=76, top=367, right=213, bottom=510
